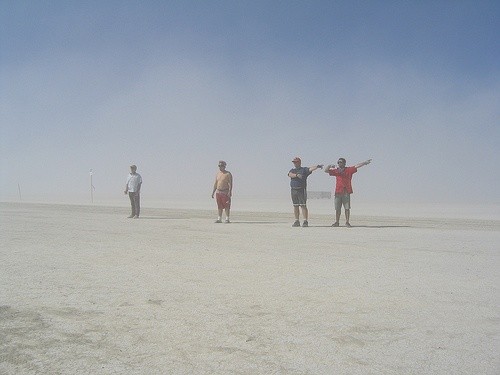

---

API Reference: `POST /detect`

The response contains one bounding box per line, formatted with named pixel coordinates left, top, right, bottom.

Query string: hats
left=292, top=157, right=301, bottom=162
left=339, top=157, right=346, bottom=162
left=217, top=161, right=227, bottom=167
left=129, top=165, right=137, bottom=170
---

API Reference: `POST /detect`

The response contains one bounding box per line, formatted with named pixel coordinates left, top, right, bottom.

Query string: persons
left=211, top=161, right=232, bottom=223
left=124, top=165, right=142, bottom=219
left=288, top=157, right=324, bottom=227
left=325, top=158, right=374, bottom=228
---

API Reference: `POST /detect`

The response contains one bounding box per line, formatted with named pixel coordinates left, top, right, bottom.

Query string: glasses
left=337, top=161, right=343, bottom=164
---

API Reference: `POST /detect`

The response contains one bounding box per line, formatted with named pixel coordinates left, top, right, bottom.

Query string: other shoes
left=128, top=214, right=139, bottom=219
left=214, top=220, right=223, bottom=223
left=332, top=222, right=340, bottom=227
left=292, top=220, right=300, bottom=227
left=225, top=220, right=230, bottom=223
left=345, top=222, right=351, bottom=227
left=301, top=220, right=308, bottom=227
